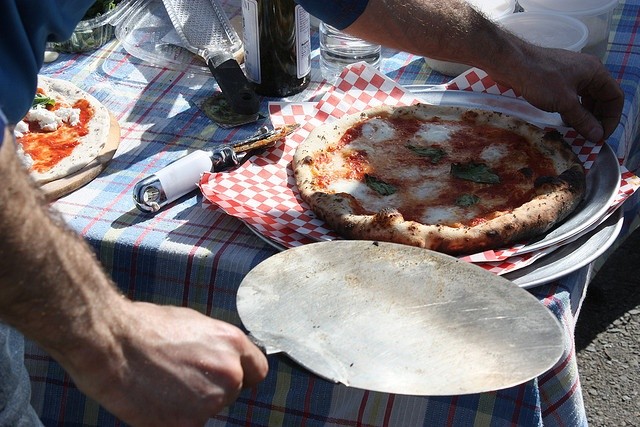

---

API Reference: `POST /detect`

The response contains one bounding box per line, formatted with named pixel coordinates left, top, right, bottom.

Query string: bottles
left=319, top=17, right=381, bottom=84
left=241, top=1, right=310, bottom=95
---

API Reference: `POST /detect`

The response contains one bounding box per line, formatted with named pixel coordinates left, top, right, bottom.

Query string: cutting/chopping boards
left=22, top=109, right=120, bottom=202
left=235, top=240, right=567, bottom=397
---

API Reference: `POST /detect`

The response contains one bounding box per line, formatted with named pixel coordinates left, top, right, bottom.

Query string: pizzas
left=14, top=75, right=110, bottom=185
left=292, top=103, right=586, bottom=256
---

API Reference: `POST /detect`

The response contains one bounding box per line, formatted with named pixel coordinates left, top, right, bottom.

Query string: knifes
left=132, top=124, right=302, bottom=214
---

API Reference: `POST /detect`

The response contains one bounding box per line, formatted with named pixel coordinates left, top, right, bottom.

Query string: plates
left=238, top=195, right=624, bottom=288
left=398, top=92, right=620, bottom=253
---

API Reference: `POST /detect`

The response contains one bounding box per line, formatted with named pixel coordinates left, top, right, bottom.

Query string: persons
left=1, top=0, right=625, bottom=427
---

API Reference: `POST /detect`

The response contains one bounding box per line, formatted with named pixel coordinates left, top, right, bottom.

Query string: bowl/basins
left=493, top=11, right=589, bottom=54
left=517, top=0, right=620, bottom=58
left=423, top=0, right=516, bottom=76
